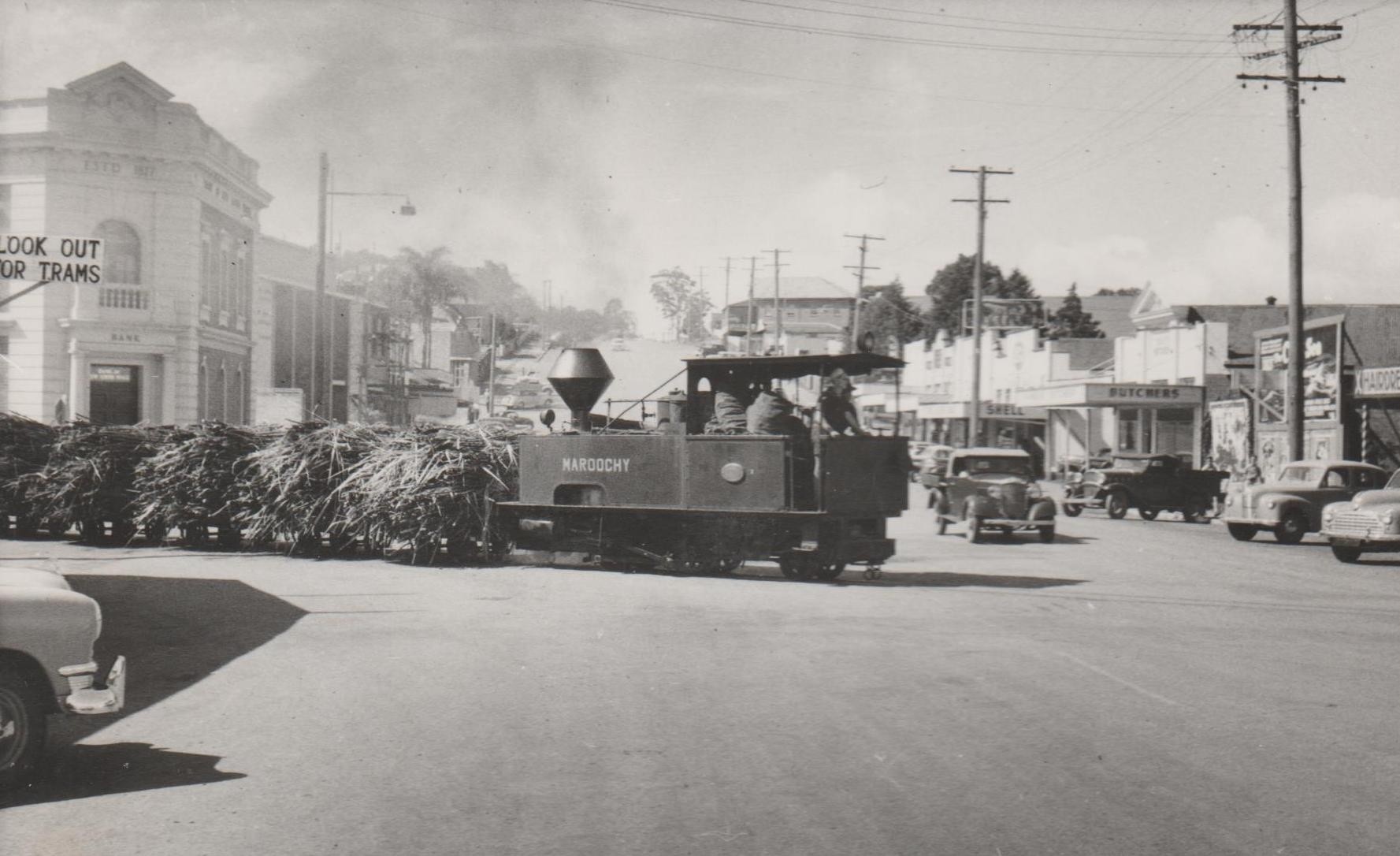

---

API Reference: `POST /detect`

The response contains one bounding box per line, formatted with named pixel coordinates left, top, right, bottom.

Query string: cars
left=1063, top=450, right=1230, bottom=523
left=1, top=548, right=129, bottom=794
left=1320, top=468, right=1400, bottom=564
left=921, top=444, right=1058, bottom=545
left=1225, top=459, right=1393, bottom=545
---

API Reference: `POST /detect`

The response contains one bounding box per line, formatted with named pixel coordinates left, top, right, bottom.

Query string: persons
left=820, top=379, right=872, bottom=436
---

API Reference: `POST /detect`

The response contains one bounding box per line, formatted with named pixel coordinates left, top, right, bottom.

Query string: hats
left=842, top=382, right=857, bottom=392
left=826, top=368, right=844, bottom=383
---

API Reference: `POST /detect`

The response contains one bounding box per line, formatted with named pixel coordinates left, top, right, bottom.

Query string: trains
left=0, top=342, right=914, bottom=585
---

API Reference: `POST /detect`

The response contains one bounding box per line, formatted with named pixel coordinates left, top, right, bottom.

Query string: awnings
left=884, top=395, right=1045, bottom=449
left=1351, top=364, right=1399, bottom=484
left=1016, top=382, right=1205, bottom=485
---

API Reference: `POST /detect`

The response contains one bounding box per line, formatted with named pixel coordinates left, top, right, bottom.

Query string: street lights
left=307, top=151, right=420, bottom=435
